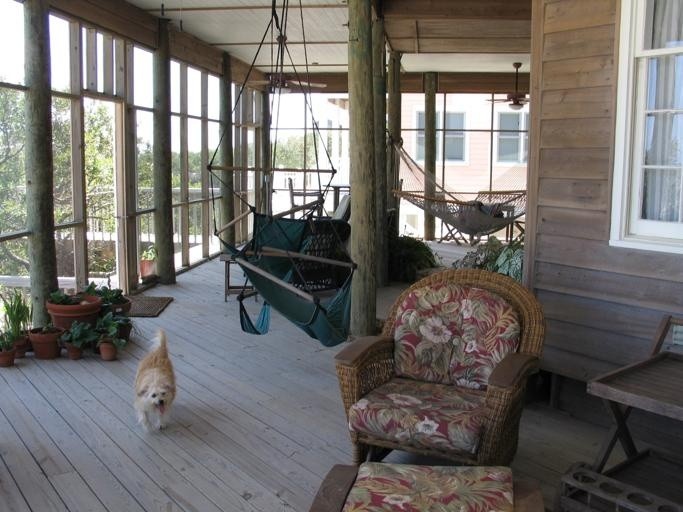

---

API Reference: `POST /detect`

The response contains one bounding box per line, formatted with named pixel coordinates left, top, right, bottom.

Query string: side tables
left=220, top=248, right=259, bottom=303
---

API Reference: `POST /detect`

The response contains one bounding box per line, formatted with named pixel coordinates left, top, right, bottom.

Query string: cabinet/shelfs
left=558, top=313, right=683, bottom=512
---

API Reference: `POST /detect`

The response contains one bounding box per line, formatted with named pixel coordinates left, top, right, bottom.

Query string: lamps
left=509, top=104, right=523, bottom=110
left=271, top=87, right=291, bottom=96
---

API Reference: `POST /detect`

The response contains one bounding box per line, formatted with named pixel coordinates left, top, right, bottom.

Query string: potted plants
left=0, top=281, right=133, bottom=368
left=139, top=246, right=160, bottom=279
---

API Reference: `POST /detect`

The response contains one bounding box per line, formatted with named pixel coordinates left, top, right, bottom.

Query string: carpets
left=115, top=295, right=173, bottom=318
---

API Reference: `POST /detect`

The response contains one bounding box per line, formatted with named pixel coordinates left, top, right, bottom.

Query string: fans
left=485, top=62, right=531, bottom=105
left=247, top=35, right=328, bottom=96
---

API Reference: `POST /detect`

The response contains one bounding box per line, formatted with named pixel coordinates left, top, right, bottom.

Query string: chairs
left=308, top=460, right=545, bottom=512
left=333, top=268, right=547, bottom=466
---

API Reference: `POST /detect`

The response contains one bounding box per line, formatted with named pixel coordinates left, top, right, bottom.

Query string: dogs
left=134, top=328, right=177, bottom=436
